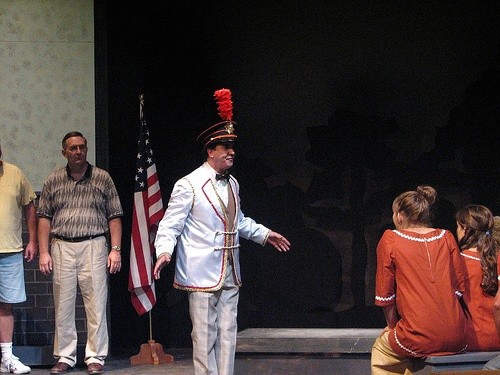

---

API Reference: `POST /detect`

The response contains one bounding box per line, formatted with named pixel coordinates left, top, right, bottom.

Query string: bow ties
left=216, top=171, right=230, bottom=181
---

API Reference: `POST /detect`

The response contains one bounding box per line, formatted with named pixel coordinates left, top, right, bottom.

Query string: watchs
left=111, top=245, right=121, bottom=252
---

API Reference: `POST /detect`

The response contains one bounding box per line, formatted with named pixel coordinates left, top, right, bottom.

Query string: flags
left=129, top=95, right=167, bottom=318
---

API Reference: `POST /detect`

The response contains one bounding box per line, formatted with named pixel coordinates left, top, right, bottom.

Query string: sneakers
left=0, top=356, right=31, bottom=374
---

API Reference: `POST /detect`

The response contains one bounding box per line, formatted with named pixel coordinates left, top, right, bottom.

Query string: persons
left=153, top=88, right=292, bottom=375
left=37, top=130, right=125, bottom=374
left=454, top=202, right=500, bottom=351
left=0, top=145, right=39, bottom=374
left=370, top=184, right=469, bottom=375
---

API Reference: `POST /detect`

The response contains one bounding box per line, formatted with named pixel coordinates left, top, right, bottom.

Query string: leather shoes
left=49, top=362, right=74, bottom=375
left=87, top=363, right=104, bottom=375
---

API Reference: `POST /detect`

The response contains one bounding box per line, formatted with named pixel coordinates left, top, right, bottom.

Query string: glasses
left=69, top=144, right=87, bottom=151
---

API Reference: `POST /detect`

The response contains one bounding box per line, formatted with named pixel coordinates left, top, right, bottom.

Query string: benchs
left=409, top=350, right=500, bottom=375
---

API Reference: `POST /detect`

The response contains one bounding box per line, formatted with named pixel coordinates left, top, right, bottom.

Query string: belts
left=53, top=233, right=105, bottom=242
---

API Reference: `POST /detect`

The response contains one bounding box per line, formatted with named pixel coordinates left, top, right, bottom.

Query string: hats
left=196, top=121, right=240, bottom=148
